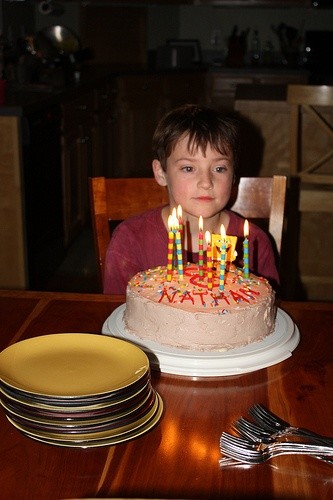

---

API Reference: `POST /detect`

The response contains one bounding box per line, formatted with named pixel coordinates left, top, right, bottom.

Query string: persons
left=102, top=103, right=284, bottom=295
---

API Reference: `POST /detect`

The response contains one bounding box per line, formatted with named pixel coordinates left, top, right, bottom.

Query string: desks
left=231, top=83, right=333, bottom=301
left=0, top=290, right=333, bottom=500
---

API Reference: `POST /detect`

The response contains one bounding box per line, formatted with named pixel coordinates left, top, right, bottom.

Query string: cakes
left=122, top=261, right=275, bottom=352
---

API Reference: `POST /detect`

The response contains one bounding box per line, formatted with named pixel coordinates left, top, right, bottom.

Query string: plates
left=0, top=333, right=165, bottom=448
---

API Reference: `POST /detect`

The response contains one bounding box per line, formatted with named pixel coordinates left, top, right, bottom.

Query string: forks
left=219, top=403, right=333, bottom=468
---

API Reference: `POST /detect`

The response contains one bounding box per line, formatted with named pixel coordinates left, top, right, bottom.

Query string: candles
left=166, top=203, right=251, bottom=290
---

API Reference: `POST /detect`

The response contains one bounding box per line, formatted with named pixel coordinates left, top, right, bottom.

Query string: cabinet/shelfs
left=117, top=61, right=305, bottom=177
left=6, top=65, right=116, bottom=248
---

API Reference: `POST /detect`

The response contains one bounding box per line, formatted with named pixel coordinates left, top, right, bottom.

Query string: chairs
left=285, top=85, right=333, bottom=301
left=90, top=176, right=286, bottom=288
